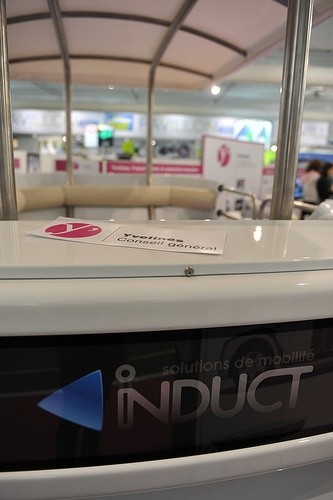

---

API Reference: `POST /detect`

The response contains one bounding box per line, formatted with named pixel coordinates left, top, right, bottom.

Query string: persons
left=316, top=163, right=333, bottom=202
left=300, top=160, right=322, bottom=205
